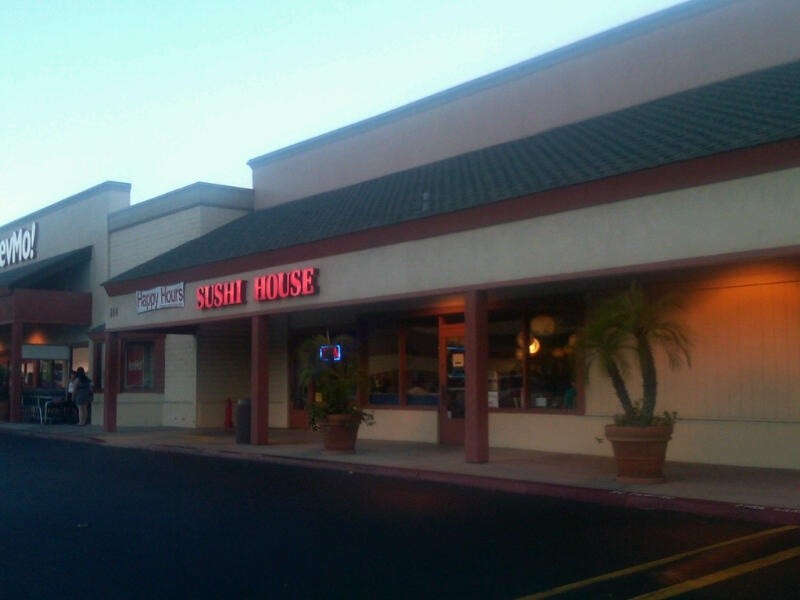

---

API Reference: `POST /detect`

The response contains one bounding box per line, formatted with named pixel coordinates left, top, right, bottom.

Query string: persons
left=72, top=367, right=91, bottom=427
left=509, top=361, right=520, bottom=409
left=68, top=372, right=78, bottom=423
left=543, top=383, right=563, bottom=403
left=561, top=379, right=575, bottom=409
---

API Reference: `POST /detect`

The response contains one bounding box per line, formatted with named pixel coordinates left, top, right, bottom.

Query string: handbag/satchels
left=89, top=384, right=94, bottom=395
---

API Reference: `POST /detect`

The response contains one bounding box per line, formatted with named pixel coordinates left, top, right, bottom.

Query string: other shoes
left=75, top=424, right=83, bottom=426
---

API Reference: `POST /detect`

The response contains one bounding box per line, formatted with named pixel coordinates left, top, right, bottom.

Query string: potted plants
left=577, top=277, right=693, bottom=478
left=296, top=330, right=375, bottom=449
